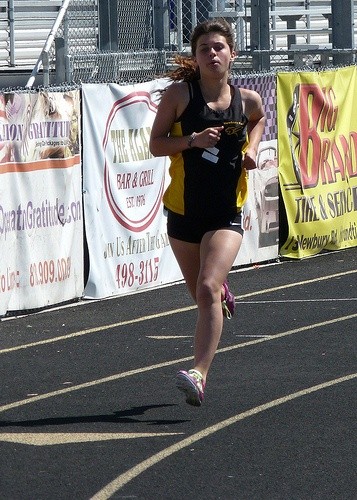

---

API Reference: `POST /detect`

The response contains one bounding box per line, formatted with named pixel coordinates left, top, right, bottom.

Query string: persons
left=148, top=22, right=266, bottom=407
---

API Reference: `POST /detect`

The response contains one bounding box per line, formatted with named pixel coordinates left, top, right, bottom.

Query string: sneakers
left=220, top=280, right=235, bottom=320
left=175, top=368, right=206, bottom=407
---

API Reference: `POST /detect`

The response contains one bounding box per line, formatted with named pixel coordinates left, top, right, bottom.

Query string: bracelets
left=188, top=132, right=198, bottom=147
